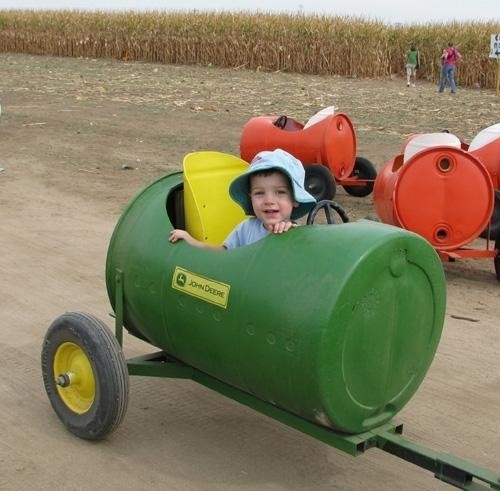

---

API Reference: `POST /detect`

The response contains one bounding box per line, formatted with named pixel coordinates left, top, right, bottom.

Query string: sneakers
left=406, top=81, right=416, bottom=87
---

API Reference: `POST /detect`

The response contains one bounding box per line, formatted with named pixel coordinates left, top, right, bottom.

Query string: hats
left=229, top=149, right=316, bottom=219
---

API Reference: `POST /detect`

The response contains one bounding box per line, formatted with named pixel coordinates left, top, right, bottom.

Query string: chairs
left=181, top=149, right=252, bottom=248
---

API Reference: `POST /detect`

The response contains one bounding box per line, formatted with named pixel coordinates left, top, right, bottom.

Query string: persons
left=405, top=46, right=421, bottom=87
left=169, top=149, right=318, bottom=249
left=437, top=42, right=461, bottom=94
